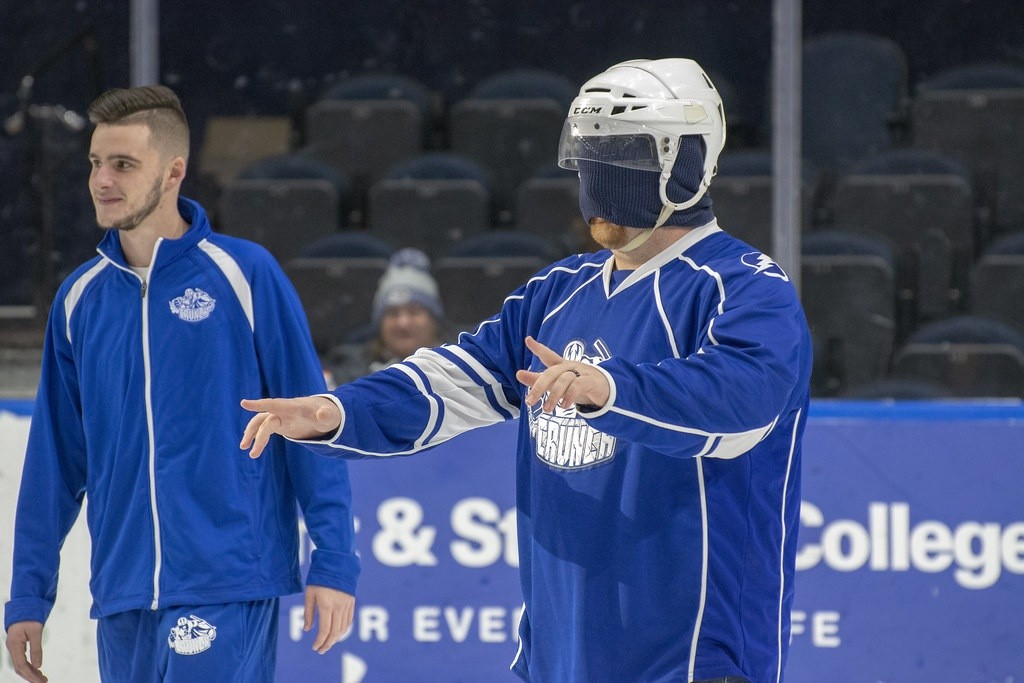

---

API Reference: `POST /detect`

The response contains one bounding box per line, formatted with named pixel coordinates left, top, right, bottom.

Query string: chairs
left=214, top=35, right=1024, bottom=399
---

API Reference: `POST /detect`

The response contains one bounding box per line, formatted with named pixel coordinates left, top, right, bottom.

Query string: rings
left=277, top=414, right=282, bottom=426
left=568, top=368, right=582, bottom=377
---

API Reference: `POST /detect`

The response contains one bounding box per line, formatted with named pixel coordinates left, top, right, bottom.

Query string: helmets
left=568, top=59, right=726, bottom=183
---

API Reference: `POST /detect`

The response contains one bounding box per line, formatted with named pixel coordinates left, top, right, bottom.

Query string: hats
left=371, top=248, right=444, bottom=326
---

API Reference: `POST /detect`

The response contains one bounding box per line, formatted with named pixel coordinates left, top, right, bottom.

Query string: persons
left=368, top=246, right=447, bottom=380
left=239, top=59, right=814, bottom=683
left=5, top=86, right=361, bottom=683
